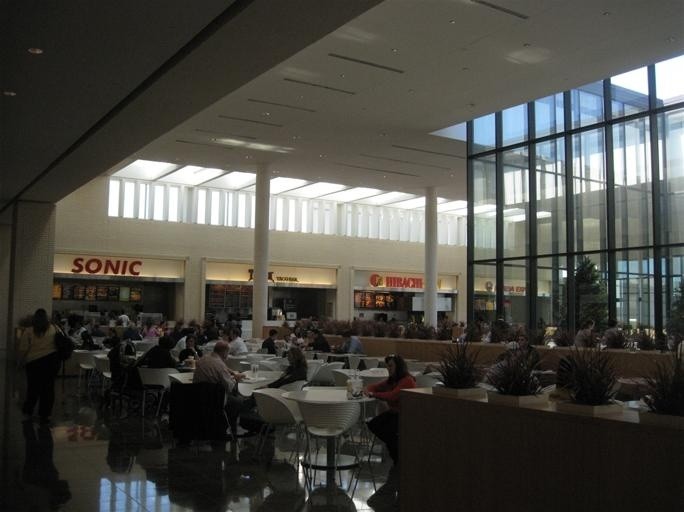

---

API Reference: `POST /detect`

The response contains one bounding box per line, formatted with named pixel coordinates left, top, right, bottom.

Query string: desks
left=283, top=390, right=367, bottom=487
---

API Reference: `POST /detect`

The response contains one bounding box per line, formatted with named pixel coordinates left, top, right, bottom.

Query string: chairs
left=170, top=384, right=227, bottom=459
left=46, top=322, right=660, bottom=439
left=257, top=392, right=303, bottom=466
left=300, top=403, right=368, bottom=497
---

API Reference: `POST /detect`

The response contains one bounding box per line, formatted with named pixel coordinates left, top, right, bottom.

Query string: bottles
left=345, top=373, right=362, bottom=399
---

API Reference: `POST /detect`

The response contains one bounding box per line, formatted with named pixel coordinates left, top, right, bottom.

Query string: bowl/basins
left=184, top=359, right=195, bottom=368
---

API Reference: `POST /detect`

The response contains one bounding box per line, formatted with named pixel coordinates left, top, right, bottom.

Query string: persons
left=15, top=308, right=623, bottom=467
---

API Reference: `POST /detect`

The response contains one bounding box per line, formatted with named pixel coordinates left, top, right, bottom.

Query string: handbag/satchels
left=53, top=330, right=73, bottom=359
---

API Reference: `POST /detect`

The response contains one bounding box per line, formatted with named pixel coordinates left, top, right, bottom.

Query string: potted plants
left=635, top=354, right=681, bottom=428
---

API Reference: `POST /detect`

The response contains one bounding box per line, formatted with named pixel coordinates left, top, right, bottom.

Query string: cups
left=250, top=364, right=258, bottom=378
left=188, top=356, right=194, bottom=359
